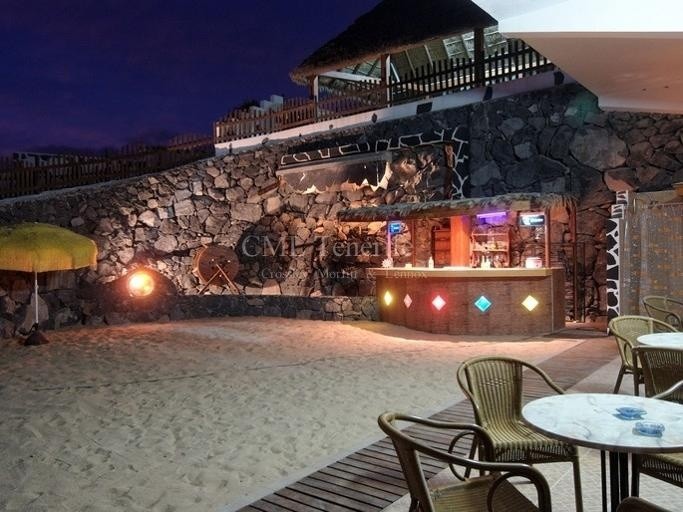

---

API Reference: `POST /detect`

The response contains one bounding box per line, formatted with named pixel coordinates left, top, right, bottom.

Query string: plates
left=616, top=407, right=647, bottom=420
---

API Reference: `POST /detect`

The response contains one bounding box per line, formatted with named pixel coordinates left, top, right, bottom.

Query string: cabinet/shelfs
left=432, top=229, right=451, bottom=268
left=469, top=232, right=510, bottom=267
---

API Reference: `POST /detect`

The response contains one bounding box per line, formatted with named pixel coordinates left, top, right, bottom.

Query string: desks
left=519, top=393, right=683, bottom=512
left=636, top=333, right=683, bottom=350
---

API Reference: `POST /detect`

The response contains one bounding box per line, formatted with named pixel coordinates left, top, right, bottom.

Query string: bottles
left=428, top=256, right=434, bottom=268
left=481, top=256, right=490, bottom=269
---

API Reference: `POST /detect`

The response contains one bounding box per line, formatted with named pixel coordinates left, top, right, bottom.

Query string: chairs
left=380, top=409, right=555, bottom=512
left=453, top=354, right=588, bottom=510
left=642, top=295, right=683, bottom=333
left=606, top=313, right=682, bottom=397
left=631, top=346, right=683, bottom=405
left=615, top=497, right=670, bottom=512
left=630, top=449, right=683, bottom=499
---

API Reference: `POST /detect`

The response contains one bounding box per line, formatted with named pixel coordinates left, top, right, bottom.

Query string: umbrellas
left=0, top=220, right=97, bottom=323
